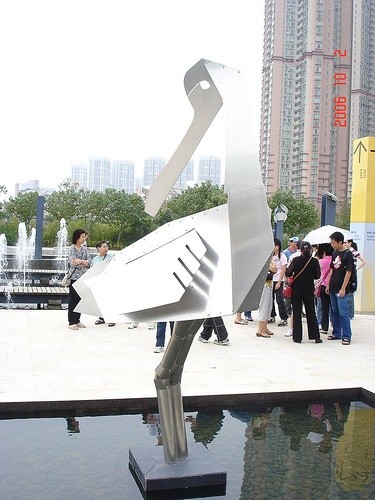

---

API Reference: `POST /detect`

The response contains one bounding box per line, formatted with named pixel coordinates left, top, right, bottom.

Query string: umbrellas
left=302, top=225, right=355, bottom=246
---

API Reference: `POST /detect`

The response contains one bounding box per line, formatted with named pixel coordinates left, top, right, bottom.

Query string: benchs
left=0, top=256, right=70, bottom=296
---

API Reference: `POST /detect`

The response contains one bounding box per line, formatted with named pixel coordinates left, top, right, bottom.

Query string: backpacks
left=333, top=249, right=357, bottom=293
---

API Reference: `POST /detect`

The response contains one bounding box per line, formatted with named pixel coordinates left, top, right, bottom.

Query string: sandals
left=108, top=323, right=115, bottom=326
left=342, top=339, right=350, bottom=345
left=327, top=334, right=341, bottom=340
left=95, top=317, right=105, bottom=324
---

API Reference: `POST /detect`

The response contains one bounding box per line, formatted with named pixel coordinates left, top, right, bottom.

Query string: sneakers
left=198, top=336, right=208, bottom=343
left=213, top=338, right=230, bottom=345
left=154, top=346, right=165, bottom=353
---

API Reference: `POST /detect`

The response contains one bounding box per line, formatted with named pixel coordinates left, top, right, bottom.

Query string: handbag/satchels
left=266, top=270, right=273, bottom=280
left=62, top=274, right=71, bottom=287
left=283, top=287, right=292, bottom=299
left=314, top=282, right=321, bottom=297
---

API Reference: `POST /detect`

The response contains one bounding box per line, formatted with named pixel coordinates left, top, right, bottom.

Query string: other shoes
left=245, top=316, right=253, bottom=321
left=235, top=319, right=248, bottom=325
left=148, top=323, right=156, bottom=329
left=265, top=329, right=273, bottom=335
left=69, top=324, right=79, bottom=330
left=76, top=322, right=86, bottom=328
left=319, top=329, right=327, bottom=334
left=256, top=329, right=271, bottom=338
left=278, top=320, right=288, bottom=325
left=285, top=329, right=293, bottom=336
left=268, top=317, right=275, bottom=322
left=128, top=323, right=138, bottom=328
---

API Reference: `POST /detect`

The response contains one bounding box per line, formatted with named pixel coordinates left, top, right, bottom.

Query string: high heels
left=294, top=340, right=301, bottom=343
left=315, top=338, right=322, bottom=343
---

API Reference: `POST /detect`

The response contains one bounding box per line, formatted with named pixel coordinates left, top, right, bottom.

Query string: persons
left=65, top=399, right=353, bottom=454
left=234, top=237, right=366, bottom=345
left=69, top=230, right=229, bottom=354
